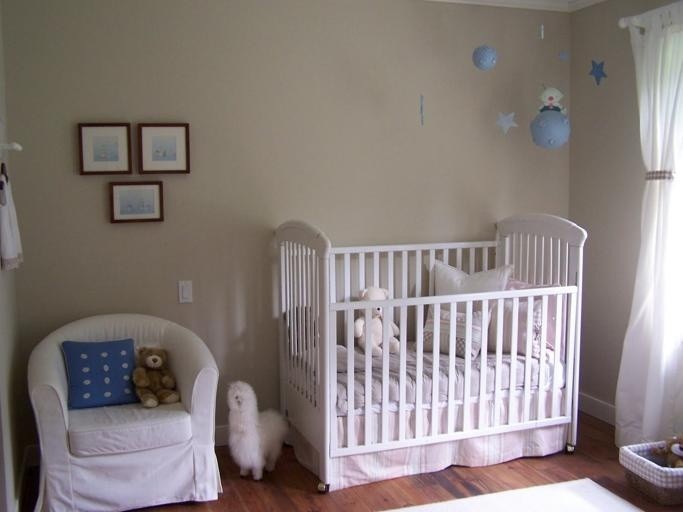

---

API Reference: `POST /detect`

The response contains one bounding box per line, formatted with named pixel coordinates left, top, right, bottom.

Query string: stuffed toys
left=651, top=436, right=683, bottom=467
left=133, top=347, right=181, bottom=409
left=355, top=288, right=400, bottom=359
left=227, top=380, right=290, bottom=480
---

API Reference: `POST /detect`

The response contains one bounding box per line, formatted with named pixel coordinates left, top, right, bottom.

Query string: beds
left=271, top=211, right=588, bottom=496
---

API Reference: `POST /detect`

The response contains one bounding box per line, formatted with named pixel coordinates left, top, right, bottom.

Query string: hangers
left=0, top=162, right=8, bottom=191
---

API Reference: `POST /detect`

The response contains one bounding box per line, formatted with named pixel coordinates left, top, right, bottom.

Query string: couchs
left=25, top=312, right=223, bottom=511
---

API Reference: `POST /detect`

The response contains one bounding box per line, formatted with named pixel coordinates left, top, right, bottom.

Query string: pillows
left=430, top=258, right=516, bottom=311
left=60, top=338, right=138, bottom=411
left=488, top=279, right=565, bottom=362
left=413, top=302, right=492, bottom=361
left=488, top=298, right=544, bottom=360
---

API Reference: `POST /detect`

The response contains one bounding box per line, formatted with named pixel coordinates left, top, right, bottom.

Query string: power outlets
left=178, top=281, right=197, bottom=310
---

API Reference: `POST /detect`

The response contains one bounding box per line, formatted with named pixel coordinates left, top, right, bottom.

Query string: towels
left=0, top=162, right=25, bottom=271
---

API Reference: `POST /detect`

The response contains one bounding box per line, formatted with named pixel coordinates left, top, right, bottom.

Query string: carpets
left=382, top=478, right=643, bottom=512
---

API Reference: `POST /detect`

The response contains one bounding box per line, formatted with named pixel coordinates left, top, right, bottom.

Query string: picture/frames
left=79, top=122, right=132, bottom=175
left=137, top=122, right=190, bottom=174
left=109, top=180, right=164, bottom=223
